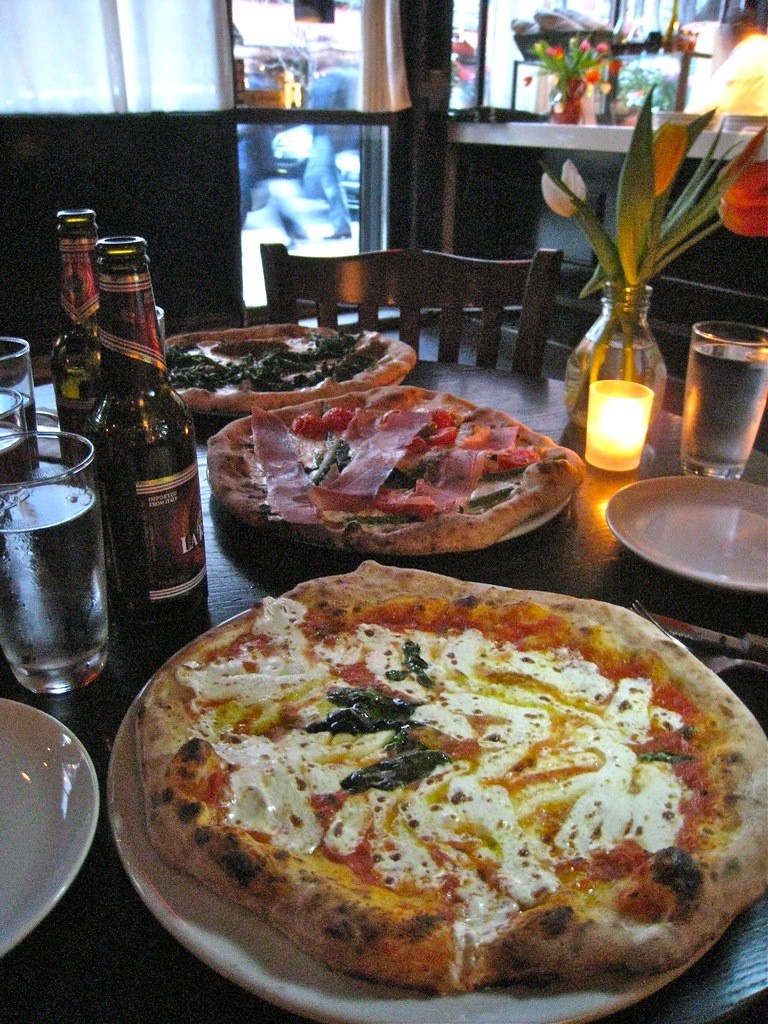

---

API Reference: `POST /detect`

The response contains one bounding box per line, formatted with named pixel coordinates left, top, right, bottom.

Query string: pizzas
left=206, top=385, right=586, bottom=554
left=135, top=558, right=768, bottom=994
left=163, top=323, right=416, bottom=414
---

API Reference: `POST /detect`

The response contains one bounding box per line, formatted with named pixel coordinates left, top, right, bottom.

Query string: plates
left=192, top=374, right=407, bottom=417
left=1, top=697, right=99, bottom=955
left=607, top=475, right=766, bottom=590
left=110, top=581, right=733, bottom=1024
left=206, top=467, right=573, bottom=550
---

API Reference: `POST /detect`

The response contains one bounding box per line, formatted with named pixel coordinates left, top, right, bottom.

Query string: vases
left=564, top=285, right=666, bottom=435
left=548, top=82, right=588, bottom=125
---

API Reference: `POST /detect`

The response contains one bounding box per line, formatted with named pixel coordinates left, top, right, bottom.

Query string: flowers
left=537, top=80, right=768, bottom=419
left=519, top=33, right=621, bottom=105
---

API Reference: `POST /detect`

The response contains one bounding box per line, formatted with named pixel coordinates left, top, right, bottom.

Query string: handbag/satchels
left=248, top=184, right=270, bottom=211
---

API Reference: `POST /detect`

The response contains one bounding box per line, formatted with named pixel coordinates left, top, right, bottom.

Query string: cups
left=0, top=484, right=111, bottom=694
left=584, top=380, right=655, bottom=471
left=0, top=389, right=32, bottom=476
left=0, top=432, right=109, bottom=490
left=0, top=337, right=39, bottom=472
left=681, top=320, right=768, bottom=479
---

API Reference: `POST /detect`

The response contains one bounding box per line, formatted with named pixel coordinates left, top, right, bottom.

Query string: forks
left=631, top=600, right=768, bottom=675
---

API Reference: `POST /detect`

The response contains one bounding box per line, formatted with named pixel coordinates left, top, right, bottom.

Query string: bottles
left=50, top=210, right=104, bottom=465
left=82, top=236, right=209, bottom=625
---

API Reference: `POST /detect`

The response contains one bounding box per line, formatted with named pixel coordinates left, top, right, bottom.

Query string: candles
left=584, top=380, right=655, bottom=470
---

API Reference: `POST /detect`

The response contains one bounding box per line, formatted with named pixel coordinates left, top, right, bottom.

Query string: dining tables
left=0, top=361, right=768, bottom=1024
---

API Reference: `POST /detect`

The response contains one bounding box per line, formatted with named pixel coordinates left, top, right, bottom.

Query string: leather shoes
left=325, top=232, right=351, bottom=238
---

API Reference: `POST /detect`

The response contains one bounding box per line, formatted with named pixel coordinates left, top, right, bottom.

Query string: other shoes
left=287, top=238, right=311, bottom=250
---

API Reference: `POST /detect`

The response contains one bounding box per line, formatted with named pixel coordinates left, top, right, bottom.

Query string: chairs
left=406, top=247, right=564, bottom=376
left=258, top=239, right=403, bottom=340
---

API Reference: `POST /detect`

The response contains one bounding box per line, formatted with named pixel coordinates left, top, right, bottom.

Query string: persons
left=301, top=49, right=361, bottom=240
left=235, top=59, right=311, bottom=252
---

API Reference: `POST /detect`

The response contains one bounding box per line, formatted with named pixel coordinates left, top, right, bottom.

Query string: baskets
left=515, top=30, right=613, bottom=61
